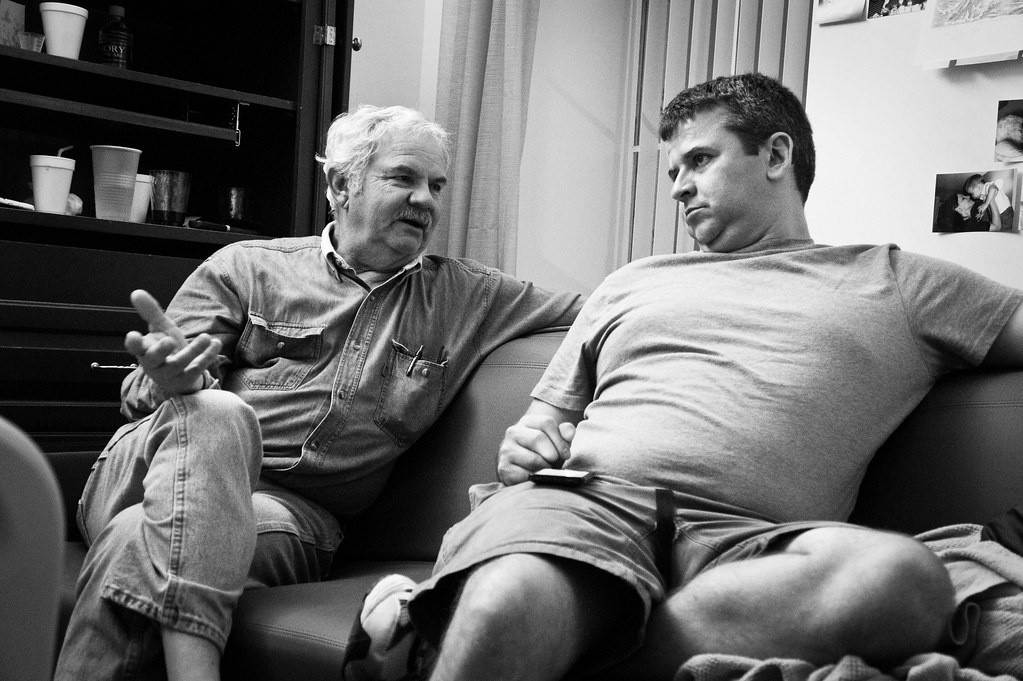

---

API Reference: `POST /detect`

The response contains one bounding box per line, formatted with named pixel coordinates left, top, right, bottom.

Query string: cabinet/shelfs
left=0, top=0, right=318, bottom=453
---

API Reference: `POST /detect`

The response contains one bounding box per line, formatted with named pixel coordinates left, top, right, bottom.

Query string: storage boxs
left=0, top=0, right=26, bottom=48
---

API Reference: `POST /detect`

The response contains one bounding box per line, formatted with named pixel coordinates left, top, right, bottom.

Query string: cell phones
left=528, top=467, right=592, bottom=488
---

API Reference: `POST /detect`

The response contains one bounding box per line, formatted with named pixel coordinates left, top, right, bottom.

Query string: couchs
left=64, top=325, right=1023, bottom=680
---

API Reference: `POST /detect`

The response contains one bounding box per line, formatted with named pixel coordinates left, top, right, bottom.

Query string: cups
left=40, top=2, right=89, bottom=60
left=31, top=155, right=75, bottom=215
left=18, top=31, right=45, bottom=53
left=148, top=170, right=191, bottom=227
left=127, top=173, right=154, bottom=222
left=91, top=145, right=143, bottom=221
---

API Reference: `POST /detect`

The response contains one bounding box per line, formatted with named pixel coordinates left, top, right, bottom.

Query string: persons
left=53, top=107, right=587, bottom=681
left=406, top=73, right=1023, bottom=681
left=944, top=174, right=1014, bottom=232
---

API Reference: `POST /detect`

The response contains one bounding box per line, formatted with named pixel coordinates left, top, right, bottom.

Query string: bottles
left=97, top=6, right=134, bottom=69
